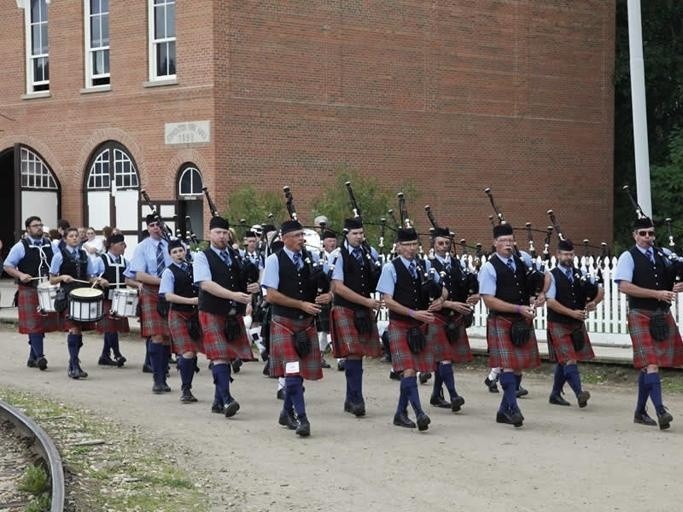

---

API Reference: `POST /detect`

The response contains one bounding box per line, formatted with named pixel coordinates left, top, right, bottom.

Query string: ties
left=646, top=250, right=653, bottom=264
left=566, top=269, right=572, bottom=283
left=353, top=248, right=363, bottom=265
left=293, top=254, right=300, bottom=270
left=221, top=251, right=230, bottom=267
left=409, top=263, right=415, bottom=279
left=156, top=243, right=166, bottom=278
left=507, top=259, right=514, bottom=272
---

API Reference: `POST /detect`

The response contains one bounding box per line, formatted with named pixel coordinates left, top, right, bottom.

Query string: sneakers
left=232, top=358, right=243, bottom=373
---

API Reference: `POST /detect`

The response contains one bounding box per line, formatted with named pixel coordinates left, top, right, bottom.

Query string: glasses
left=438, top=241, right=450, bottom=246
left=149, top=222, right=160, bottom=227
left=30, top=224, right=43, bottom=228
left=639, top=232, right=655, bottom=236
left=293, top=233, right=305, bottom=238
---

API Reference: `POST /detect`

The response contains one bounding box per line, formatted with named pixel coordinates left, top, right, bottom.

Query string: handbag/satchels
left=54, top=290, right=68, bottom=314
left=511, top=321, right=530, bottom=347
left=407, top=326, right=426, bottom=353
left=186, top=315, right=204, bottom=340
left=156, top=296, right=171, bottom=318
left=223, top=315, right=242, bottom=341
left=446, top=319, right=460, bottom=345
left=353, top=309, right=374, bottom=336
left=649, top=313, right=668, bottom=342
left=572, top=329, right=585, bottom=352
left=294, top=330, right=313, bottom=356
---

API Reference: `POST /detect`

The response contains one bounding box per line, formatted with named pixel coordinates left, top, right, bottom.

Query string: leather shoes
left=152, top=383, right=162, bottom=394
left=511, top=408, right=524, bottom=427
left=161, top=383, right=171, bottom=391
left=430, top=393, right=451, bottom=408
left=277, top=387, right=288, bottom=401
left=295, top=422, right=310, bottom=437
left=279, top=411, right=299, bottom=429
left=497, top=411, right=514, bottom=423
left=143, top=362, right=171, bottom=378
left=222, top=396, right=239, bottom=417
left=419, top=372, right=431, bottom=385
left=36, top=357, right=48, bottom=370
left=113, top=354, right=126, bottom=367
left=320, top=359, right=331, bottom=368
left=344, top=398, right=366, bottom=416
left=549, top=393, right=570, bottom=405
left=389, top=368, right=400, bottom=380
left=179, top=392, right=197, bottom=404
left=99, top=355, right=117, bottom=365
left=633, top=411, right=657, bottom=426
left=417, top=415, right=431, bottom=431
left=393, top=411, right=415, bottom=428
left=69, top=365, right=88, bottom=378
left=212, top=398, right=223, bottom=413
left=578, top=391, right=590, bottom=408
left=338, top=360, right=346, bottom=370
left=656, top=405, right=672, bottom=429
left=28, top=357, right=37, bottom=367
left=515, top=385, right=528, bottom=397
left=450, top=397, right=464, bottom=411
left=484, top=377, right=499, bottom=393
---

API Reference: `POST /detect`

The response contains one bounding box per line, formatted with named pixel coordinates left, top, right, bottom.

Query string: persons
left=613, top=218, right=683, bottom=430
left=261, top=220, right=336, bottom=436
left=260, top=296, right=274, bottom=378
left=330, top=218, right=385, bottom=416
left=542, top=241, right=604, bottom=408
left=13, top=219, right=122, bottom=252
left=122, top=230, right=171, bottom=378
left=48, top=228, right=103, bottom=379
left=425, top=227, right=481, bottom=413
left=389, top=366, right=430, bottom=384
left=92, top=234, right=131, bottom=368
left=239, top=230, right=269, bottom=362
left=313, top=231, right=345, bottom=370
left=375, top=229, right=449, bottom=431
left=192, top=216, right=261, bottom=418
left=128, top=213, right=175, bottom=394
left=478, top=224, right=551, bottom=428
left=379, top=325, right=393, bottom=363
left=156, top=239, right=199, bottom=404
left=484, top=251, right=542, bottom=398
left=207, top=357, right=244, bottom=374
left=2, top=216, right=58, bottom=370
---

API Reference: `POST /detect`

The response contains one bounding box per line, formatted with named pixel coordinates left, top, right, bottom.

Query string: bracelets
left=439, top=295, right=445, bottom=305
left=540, top=290, right=546, bottom=295
left=591, top=301, right=596, bottom=307
left=515, top=304, right=520, bottom=313
left=407, top=309, right=413, bottom=317
left=328, top=291, right=335, bottom=302
left=401, top=307, right=407, bottom=316
left=255, top=281, right=261, bottom=289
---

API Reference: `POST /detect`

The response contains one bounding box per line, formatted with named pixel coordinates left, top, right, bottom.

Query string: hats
left=558, top=239, right=574, bottom=251
left=344, top=216, right=364, bottom=229
left=397, top=227, right=418, bottom=242
left=429, top=228, right=449, bottom=239
left=280, top=220, right=302, bottom=236
left=263, top=224, right=277, bottom=234
left=323, top=231, right=337, bottom=239
left=168, top=239, right=185, bottom=255
left=209, top=217, right=229, bottom=230
left=107, top=235, right=125, bottom=243
left=245, top=230, right=260, bottom=237
left=493, top=224, right=513, bottom=238
left=146, top=213, right=162, bottom=225
left=631, top=217, right=653, bottom=230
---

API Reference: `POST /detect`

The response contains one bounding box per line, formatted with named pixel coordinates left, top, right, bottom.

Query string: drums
left=111, top=288, right=140, bottom=319
left=68, top=288, right=104, bottom=322
left=37, top=281, right=60, bottom=313
